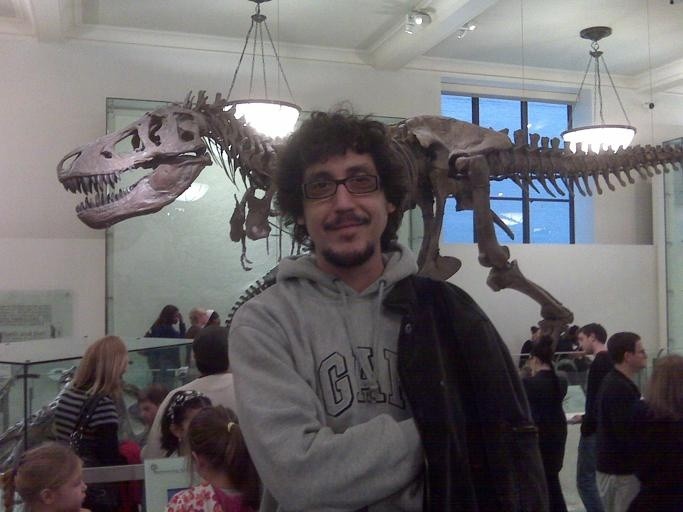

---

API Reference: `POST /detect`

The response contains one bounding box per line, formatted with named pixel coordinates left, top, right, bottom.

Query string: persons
left=522, top=333, right=570, bottom=511
left=136, top=381, right=168, bottom=426
left=625, top=353, right=681, bottom=512
left=158, top=389, right=264, bottom=511
left=0, top=440, right=94, bottom=512
left=592, top=329, right=649, bottom=511
left=573, top=322, right=614, bottom=511
left=139, top=326, right=235, bottom=461
left=517, top=323, right=594, bottom=394
left=142, top=304, right=221, bottom=385
left=52, top=335, right=132, bottom=469
left=226, top=99, right=553, bottom=512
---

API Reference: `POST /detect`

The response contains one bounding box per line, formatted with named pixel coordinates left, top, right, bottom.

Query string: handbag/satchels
left=77, top=448, right=126, bottom=512
left=138, top=331, right=152, bottom=355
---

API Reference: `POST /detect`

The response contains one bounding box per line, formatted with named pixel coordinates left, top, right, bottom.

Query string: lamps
left=404, top=7, right=431, bottom=35
left=561, top=27, right=637, bottom=154
left=224, top=1, right=301, bottom=145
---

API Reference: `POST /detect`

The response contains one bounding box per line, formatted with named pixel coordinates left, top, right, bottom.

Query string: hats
left=188, top=308, right=213, bottom=328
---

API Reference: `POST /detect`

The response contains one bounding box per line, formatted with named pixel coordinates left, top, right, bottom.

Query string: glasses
left=304, top=175, right=379, bottom=199
